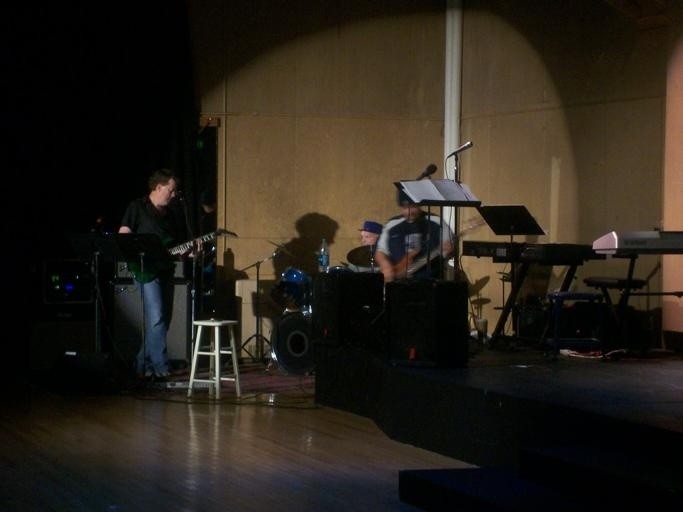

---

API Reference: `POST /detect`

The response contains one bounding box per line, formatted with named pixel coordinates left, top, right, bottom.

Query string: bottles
left=318, top=237, right=331, bottom=274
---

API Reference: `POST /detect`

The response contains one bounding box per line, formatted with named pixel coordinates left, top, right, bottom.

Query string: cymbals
left=468, top=276, right=489, bottom=297
left=472, top=298, right=491, bottom=305
left=346, top=244, right=379, bottom=268
left=267, top=238, right=300, bottom=261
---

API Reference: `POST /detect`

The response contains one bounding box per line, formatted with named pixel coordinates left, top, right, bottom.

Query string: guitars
left=128, top=228, right=238, bottom=285
left=384, top=218, right=484, bottom=279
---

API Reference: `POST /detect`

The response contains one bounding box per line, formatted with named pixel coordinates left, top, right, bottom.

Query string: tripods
left=221, top=269, right=279, bottom=370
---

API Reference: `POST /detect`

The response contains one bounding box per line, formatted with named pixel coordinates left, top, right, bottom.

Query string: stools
left=189, top=317, right=245, bottom=402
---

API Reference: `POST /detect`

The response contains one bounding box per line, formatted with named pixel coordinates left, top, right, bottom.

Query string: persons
left=347, top=221, right=382, bottom=273
left=118, top=168, right=203, bottom=381
left=374, top=181, right=457, bottom=283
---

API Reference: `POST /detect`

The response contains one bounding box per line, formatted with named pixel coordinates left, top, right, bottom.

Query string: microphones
left=415, top=162, right=438, bottom=180
left=447, top=141, right=473, bottom=158
left=264, top=237, right=298, bottom=261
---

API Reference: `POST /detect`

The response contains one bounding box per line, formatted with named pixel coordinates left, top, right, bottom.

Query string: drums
left=270, top=306, right=316, bottom=380
left=271, top=266, right=313, bottom=311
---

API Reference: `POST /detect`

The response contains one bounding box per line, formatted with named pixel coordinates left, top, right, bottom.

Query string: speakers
left=386, top=281, right=468, bottom=370
left=112, top=278, right=199, bottom=364
left=309, top=269, right=385, bottom=360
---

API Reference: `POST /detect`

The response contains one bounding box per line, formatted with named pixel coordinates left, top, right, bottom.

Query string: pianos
left=462, top=241, right=608, bottom=266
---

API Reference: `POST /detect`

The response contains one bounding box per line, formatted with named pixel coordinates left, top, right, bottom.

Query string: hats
left=397, top=191, right=414, bottom=207
left=357, top=220, right=384, bottom=235
